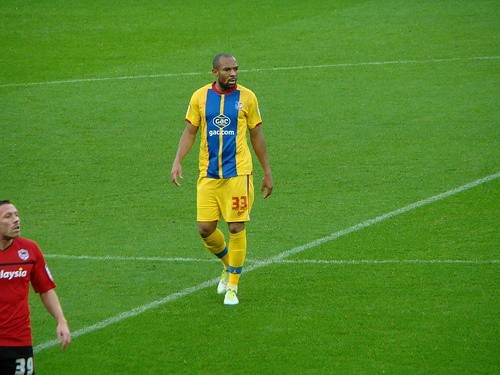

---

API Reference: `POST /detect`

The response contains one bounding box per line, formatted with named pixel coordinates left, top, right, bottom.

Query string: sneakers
left=224, top=290, right=239, bottom=304
left=216, top=269, right=230, bottom=294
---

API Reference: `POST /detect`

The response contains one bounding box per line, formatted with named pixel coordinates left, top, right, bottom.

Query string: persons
left=1, top=200, right=72, bottom=375
left=170, top=53, right=273, bottom=305
